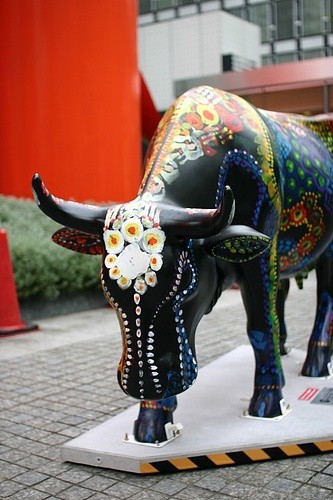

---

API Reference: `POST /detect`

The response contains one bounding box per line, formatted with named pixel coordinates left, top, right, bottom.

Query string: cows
left=29, top=85, right=333, bottom=446
